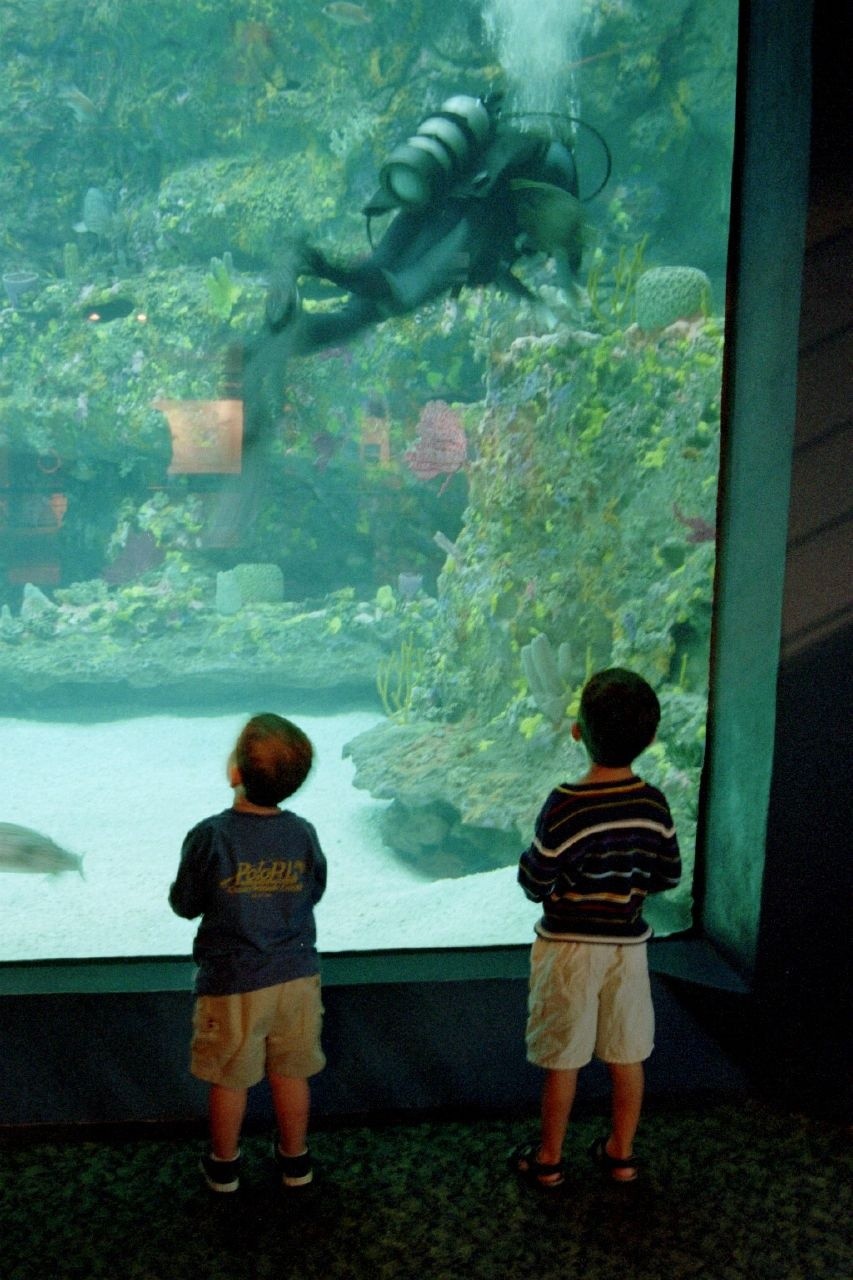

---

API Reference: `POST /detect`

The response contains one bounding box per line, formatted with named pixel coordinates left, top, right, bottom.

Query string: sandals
left=588, top=1137, right=639, bottom=1189
left=506, top=1143, right=566, bottom=1192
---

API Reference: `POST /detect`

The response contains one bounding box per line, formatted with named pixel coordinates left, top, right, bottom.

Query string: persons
left=519, top=668, right=682, bottom=1186
left=265, top=136, right=583, bottom=355
left=170, top=711, right=326, bottom=1191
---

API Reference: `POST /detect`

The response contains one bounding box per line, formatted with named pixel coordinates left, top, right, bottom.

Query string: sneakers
left=196, top=1146, right=241, bottom=1194
left=270, top=1130, right=313, bottom=1188
